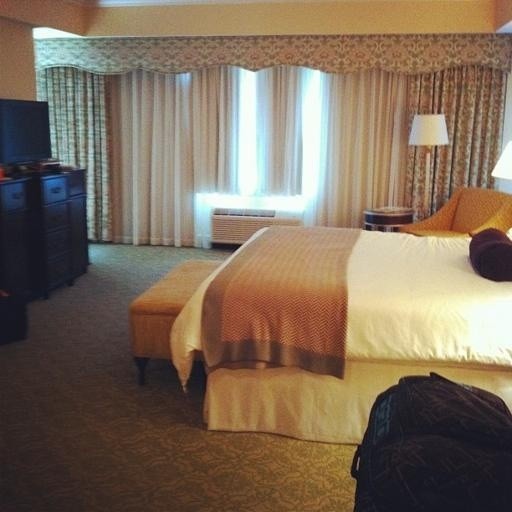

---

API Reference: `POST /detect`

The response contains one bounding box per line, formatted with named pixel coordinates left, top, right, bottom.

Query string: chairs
left=399, top=186, right=512, bottom=238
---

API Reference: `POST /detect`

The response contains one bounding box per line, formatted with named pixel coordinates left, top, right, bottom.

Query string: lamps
left=491, top=141, right=512, bottom=180
left=410, top=112, right=449, bottom=217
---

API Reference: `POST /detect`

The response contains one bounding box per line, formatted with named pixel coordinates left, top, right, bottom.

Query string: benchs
left=128, top=260, right=226, bottom=395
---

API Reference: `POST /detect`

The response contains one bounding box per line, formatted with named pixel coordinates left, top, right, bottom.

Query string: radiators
left=209, top=208, right=306, bottom=246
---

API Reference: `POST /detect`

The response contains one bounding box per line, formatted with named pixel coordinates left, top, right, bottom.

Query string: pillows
left=469, top=228, right=512, bottom=281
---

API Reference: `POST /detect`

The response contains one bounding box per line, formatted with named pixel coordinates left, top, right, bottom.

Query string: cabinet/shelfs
left=0, top=169, right=89, bottom=303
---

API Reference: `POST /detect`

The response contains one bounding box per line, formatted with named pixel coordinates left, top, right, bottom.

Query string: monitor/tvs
left=0, top=99, right=62, bottom=180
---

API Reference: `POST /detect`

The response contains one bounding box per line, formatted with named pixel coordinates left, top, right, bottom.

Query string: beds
left=170, top=225, right=512, bottom=445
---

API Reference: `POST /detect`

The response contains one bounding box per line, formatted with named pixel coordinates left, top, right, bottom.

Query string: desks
left=363, top=206, right=414, bottom=232
